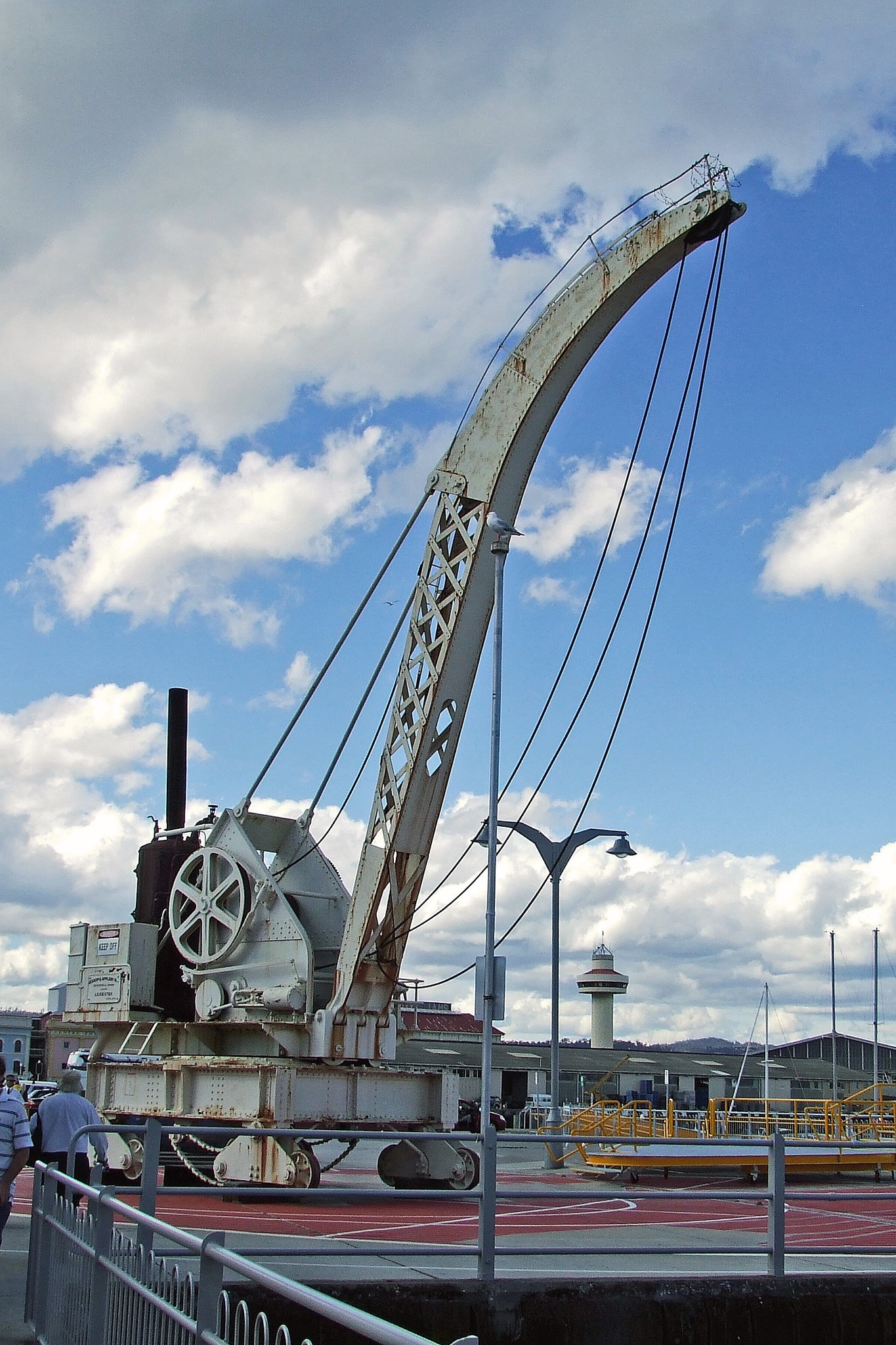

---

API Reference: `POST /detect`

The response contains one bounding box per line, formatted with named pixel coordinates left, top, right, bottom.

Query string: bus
left=64, top=1048, right=160, bottom=1095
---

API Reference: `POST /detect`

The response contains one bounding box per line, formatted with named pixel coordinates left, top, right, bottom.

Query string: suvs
left=471, top=1095, right=503, bottom=1110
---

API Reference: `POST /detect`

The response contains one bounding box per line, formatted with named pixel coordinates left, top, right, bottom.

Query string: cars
left=458, top=1099, right=508, bottom=1133
left=3, top=1078, right=59, bottom=1110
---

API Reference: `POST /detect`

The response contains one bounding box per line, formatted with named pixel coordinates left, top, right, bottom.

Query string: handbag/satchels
left=25, top=1111, right=42, bottom=1168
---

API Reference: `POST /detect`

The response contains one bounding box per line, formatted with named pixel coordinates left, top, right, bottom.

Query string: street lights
left=472, top=817, right=639, bottom=1173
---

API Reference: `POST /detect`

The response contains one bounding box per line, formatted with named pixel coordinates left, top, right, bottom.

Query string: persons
left=0, top=1054, right=34, bottom=1248
left=28, top=1071, right=109, bottom=1219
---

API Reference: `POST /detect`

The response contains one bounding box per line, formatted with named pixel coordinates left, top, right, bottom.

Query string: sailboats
left=532, top=926, right=895, bottom=1187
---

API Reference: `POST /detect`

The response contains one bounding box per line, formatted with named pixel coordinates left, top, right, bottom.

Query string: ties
left=7, top=1089, right=10, bottom=1094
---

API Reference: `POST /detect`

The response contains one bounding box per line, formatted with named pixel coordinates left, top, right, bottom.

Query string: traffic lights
left=21, top=1065, right=27, bottom=1072
left=17, top=1064, right=20, bottom=1075
left=36, top=1063, right=43, bottom=1075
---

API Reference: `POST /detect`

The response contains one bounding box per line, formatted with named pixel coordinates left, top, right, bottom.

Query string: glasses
left=6, top=1080, right=15, bottom=1082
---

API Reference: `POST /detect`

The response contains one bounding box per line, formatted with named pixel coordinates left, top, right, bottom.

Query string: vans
left=526, top=1094, right=552, bottom=1107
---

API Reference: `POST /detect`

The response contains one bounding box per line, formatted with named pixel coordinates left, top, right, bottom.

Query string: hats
left=57, top=1070, right=84, bottom=1092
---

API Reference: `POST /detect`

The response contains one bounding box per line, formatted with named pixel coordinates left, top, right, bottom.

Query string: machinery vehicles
left=584, top=1055, right=632, bottom=1107
